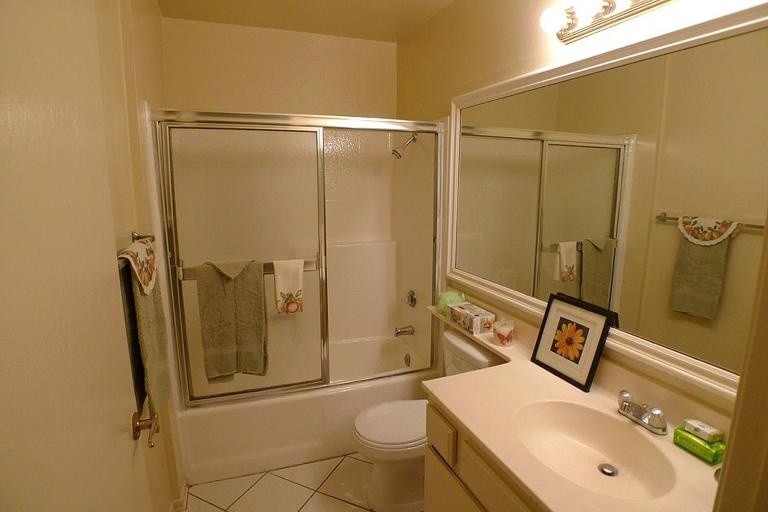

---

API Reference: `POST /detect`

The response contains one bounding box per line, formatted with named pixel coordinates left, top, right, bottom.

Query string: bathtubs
left=178, top=335, right=427, bottom=486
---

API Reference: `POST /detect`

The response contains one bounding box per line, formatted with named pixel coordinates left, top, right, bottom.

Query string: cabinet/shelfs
left=425, top=402, right=531, bottom=511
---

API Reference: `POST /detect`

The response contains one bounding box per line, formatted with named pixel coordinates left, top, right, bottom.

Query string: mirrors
left=445, top=5, right=768, bottom=405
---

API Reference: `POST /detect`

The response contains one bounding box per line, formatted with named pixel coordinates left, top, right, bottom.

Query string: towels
left=195, top=259, right=304, bottom=385
left=553, top=238, right=617, bottom=309
left=670, top=218, right=732, bottom=322
left=119, top=259, right=170, bottom=417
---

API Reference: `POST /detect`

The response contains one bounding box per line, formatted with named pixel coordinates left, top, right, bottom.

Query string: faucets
left=621, top=398, right=648, bottom=417
left=394, top=326, right=414, bottom=336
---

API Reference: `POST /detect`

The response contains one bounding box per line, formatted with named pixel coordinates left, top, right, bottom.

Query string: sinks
left=511, top=400, right=676, bottom=499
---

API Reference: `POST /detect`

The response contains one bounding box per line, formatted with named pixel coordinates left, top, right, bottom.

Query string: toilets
left=351, top=329, right=503, bottom=512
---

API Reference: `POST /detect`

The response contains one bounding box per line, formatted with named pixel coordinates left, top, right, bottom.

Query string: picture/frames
left=529, top=292, right=613, bottom=393
